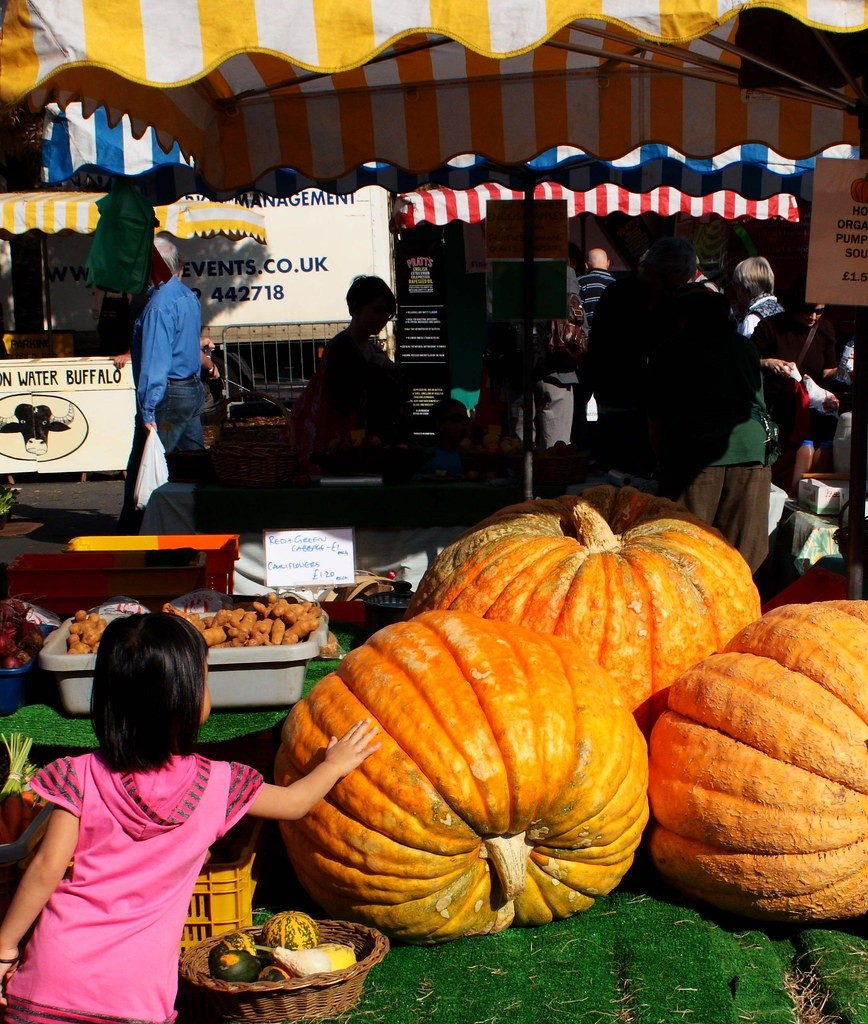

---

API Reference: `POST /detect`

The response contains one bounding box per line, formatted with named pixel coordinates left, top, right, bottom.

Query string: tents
left=0, top=0, right=868, bottom=240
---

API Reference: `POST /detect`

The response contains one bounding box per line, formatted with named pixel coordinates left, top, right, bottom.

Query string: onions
left=0, top=600, right=42, bottom=668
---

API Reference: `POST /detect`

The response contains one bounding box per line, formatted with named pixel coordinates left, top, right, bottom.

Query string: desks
left=192, top=480, right=568, bottom=533
left=769, top=501, right=843, bottom=576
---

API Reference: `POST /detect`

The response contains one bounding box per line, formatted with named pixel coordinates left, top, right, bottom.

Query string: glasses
left=798, top=306, right=826, bottom=315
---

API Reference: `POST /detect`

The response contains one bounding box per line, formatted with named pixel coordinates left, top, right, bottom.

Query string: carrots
left=0, top=732, right=46, bottom=844
left=66, top=594, right=322, bottom=654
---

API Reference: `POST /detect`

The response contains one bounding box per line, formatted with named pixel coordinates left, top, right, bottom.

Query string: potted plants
left=0, top=492, right=15, bottom=530
left=0, top=488, right=20, bottom=523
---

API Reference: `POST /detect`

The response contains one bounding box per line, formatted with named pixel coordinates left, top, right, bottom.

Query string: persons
left=0, top=613, right=383, bottom=1024
left=114, top=228, right=842, bottom=574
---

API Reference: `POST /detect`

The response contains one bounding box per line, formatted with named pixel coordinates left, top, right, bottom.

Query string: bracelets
left=0, top=946, right=22, bottom=964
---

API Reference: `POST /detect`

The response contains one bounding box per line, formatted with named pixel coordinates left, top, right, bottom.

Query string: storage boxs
left=287, top=580, right=412, bottom=626
left=60, top=533, right=240, bottom=594
left=36, top=614, right=129, bottom=716
left=181, top=817, right=265, bottom=952
left=199, top=610, right=329, bottom=710
left=0, top=625, right=54, bottom=716
left=5, top=547, right=208, bottom=614
left=798, top=478, right=849, bottom=515
left=0, top=801, right=54, bottom=922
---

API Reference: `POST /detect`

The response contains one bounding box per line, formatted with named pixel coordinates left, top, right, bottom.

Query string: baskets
left=209, top=391, right=302, bottom=486
left=179, top=920, right=390, bottom=1024
left=834, top=494, right=868, bottom=561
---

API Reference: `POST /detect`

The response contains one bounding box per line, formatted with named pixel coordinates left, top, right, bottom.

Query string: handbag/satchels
left=546, top=291, right=589, bottom=361
left=133, top=426, right=170, bottom=509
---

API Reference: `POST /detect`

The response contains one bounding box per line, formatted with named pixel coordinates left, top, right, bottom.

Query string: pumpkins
left=274, top=611, right=649, bottom=941
left=649, top=600, right=868, bottom=924
left=404, top=487, right=762, bottom=738
left=214, top=911, right=356, bottom=985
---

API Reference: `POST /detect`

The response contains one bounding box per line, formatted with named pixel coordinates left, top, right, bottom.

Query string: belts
left=170, top=376, right=192, bottom=382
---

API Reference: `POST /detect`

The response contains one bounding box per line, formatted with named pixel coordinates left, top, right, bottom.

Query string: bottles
left=792, top=440, right=814, bottom=493
left=812, top=441, right=834, bottom=475
left=834, top=336, right=855, bottom=382
